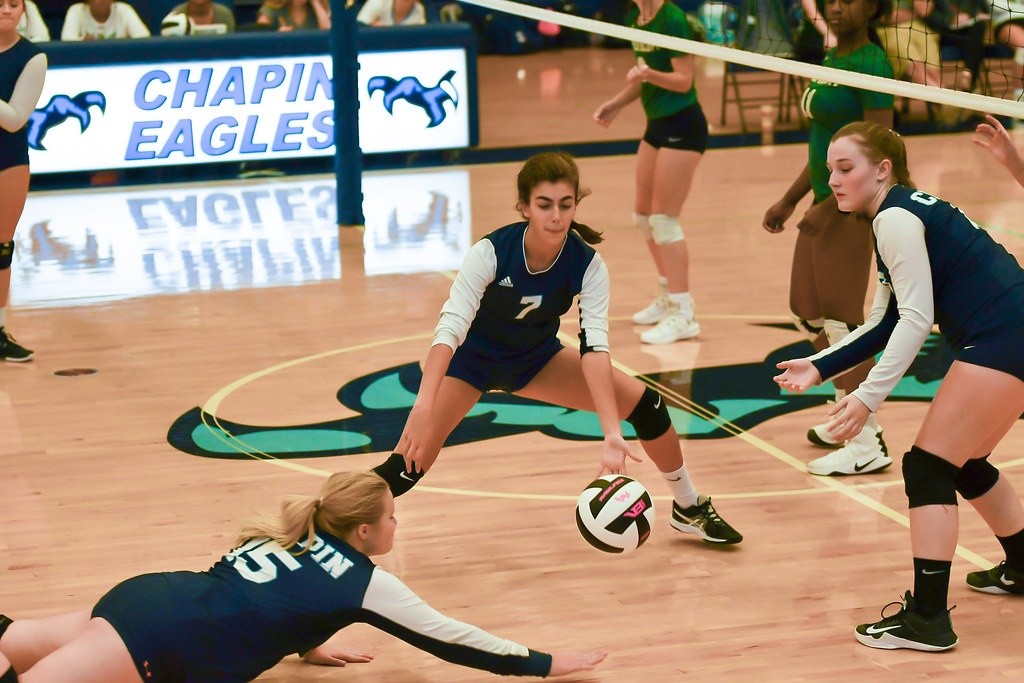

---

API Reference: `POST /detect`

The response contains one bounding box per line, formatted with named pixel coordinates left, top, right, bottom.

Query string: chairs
left=721, top=0, right=1013, bottom=135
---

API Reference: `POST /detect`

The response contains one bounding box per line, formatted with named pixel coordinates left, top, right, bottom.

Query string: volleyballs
left=573, top=473, right=657, bottom=556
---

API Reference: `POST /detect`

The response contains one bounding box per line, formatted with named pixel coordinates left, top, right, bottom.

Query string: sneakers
left=0, top=327, right=34, bottom=361
left=965, top=558, right=1024, bottom=595
left=632, top=297, right=669, bottom=324
left=671, top=496, right=743, bottom=544
left=640, top=311, right=701, bottom=345
left=808, top=423, right=891, bottom=475
left=808, top=401, right=845, bottom=446
left=854, top=590, right=959, bottom=652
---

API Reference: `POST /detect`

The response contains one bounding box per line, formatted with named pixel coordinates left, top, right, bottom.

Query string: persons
left=972, top=114, right=1024, bottom=191
left=773, top=122, right=1024, bottom=652
left=356, top=0, right=426, bottom=26
left=762, top=0, right=896, bottom=474
left=60, top=0, right=151, bottom=41
left=257, top=0, right=331, bottom=32
left=593, top=0, right=709, bottom=344
left=0, top=470, right=607, bottom=683
left=16, top=0, right=51, bottom=43
left=0, top=0, right=46, bottom=362
left=161, top=0, right=236, bottom=38
left=372, top=153, right=746, bottom=543
left=800, top=0, right=1024, bottom=120
